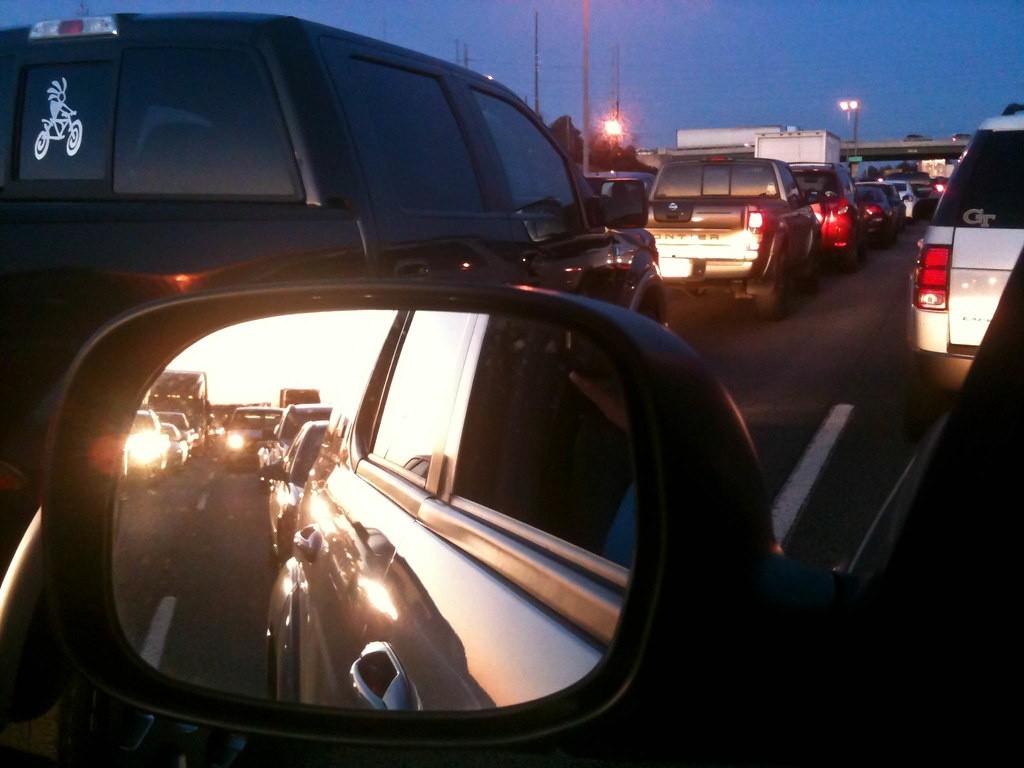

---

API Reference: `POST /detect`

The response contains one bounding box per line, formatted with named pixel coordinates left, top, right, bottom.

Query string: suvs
left=2, top=10, right=668, bottom=449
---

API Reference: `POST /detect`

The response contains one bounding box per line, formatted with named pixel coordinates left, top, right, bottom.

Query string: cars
left=111, top=301, right=642, bottom=714
left=581, top=70, right=1022, bottom=396
